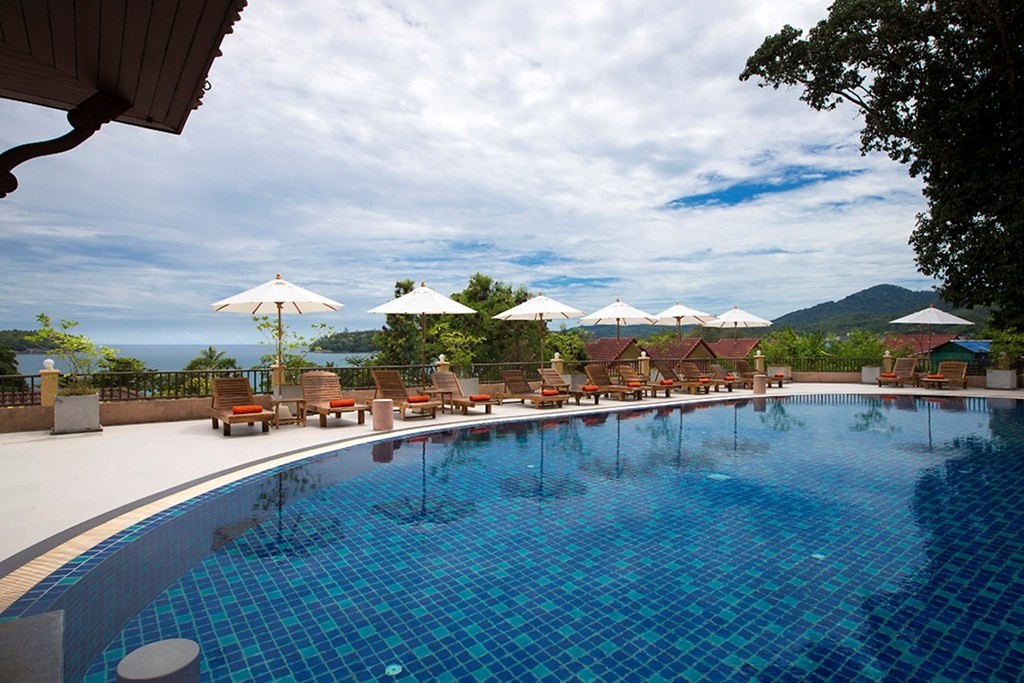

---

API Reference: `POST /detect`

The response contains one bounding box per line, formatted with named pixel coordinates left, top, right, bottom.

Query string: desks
left=742, top=371, right=767, bottom=389
left=264, top=397, right=306, bottom=428
left=913, top=371, right=936, bottom=389
left=687, top=375, right=710, bottom=393
left=420, top=390, right=453, bottom=415
left=540, top=383, right=570, bottom=403
left=619, top=378, right=648, bottom=398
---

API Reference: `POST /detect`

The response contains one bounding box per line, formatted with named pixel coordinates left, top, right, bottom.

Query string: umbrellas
left=210, top=271, right=347, bottom=391
left=580, top=298, right=774, bottom=386
left=210, top=404, right=770, bottom=563
left=491, top=290, right=588, bottom=385
left=365, top=279, right=478, bottom=389
left=888, top=303, right=977, bottom=373
left=891, top=396, right=972, bottom=458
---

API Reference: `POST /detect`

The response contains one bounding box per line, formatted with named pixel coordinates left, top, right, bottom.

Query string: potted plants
left=985, top=329, right=1024, bottom=388
left=22, top=313, right=122, bottom=434
left=757, top=329, right=798, bottom=379
left=252, top=314, right=335, bottom=415
left=429, top=322, right=486, bottom=398
left=861, top=340, right=882, bottom=383
left=544, top=324, right=593, bottom=391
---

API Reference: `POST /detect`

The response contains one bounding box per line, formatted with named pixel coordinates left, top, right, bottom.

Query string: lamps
left=554, top=352, right=560, bottom=359
left=885, top=351, right=890, bottom=355
left=641, top=351, right=646, bottom=356
left=275, top=356, right=283, bottom=366
left=43, top=359, right=55, bottom=370
left=757, top=350, right=761, bottom=355
left=439, top=354, right=445, bottom=362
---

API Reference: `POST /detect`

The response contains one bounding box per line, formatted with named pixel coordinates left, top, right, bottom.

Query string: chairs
left=923, top=360, right=969, bottom=389
left=680, top=363, right=734, bottom=392
left=875, top=357, right=920, bottom=387
left=581, top=364, right=647, bottom=400
left=493, top=369, right=570, bottom=407
left=537, top=368, right=606, bottom=406
left=615, top=366, right=682, bottom=397
left=709, top=360, right=793, bottom=389
left=654, top=362, right=703, bottom=394
left=365, top=370, right=442, bottom=420
left=429, top=371, right=498, bottom=414
left=296, top=370, right=369, bottom=427
left=207, top=378, right=276, bottom=436
